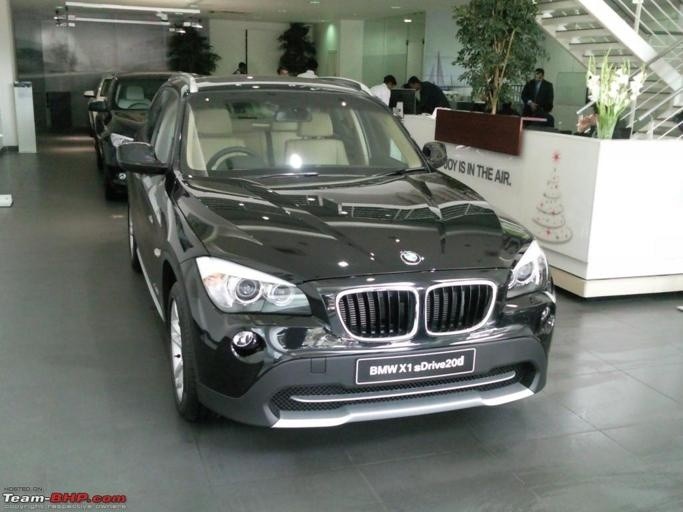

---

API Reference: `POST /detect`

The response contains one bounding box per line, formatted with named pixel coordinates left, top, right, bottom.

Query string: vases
left=596, top=117, right=617, bottom=139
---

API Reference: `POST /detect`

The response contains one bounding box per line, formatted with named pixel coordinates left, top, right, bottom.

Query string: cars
left=82, top=72, right=113, bottom=138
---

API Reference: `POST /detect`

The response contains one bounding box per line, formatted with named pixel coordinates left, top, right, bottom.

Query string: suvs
left=87, top=69, right=196, bottom=206
left=113, top=68, right=559, bottom=440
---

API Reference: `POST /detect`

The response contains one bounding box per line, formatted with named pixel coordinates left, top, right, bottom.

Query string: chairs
left=118, top=86, right=151, bottom=109
left=194, top=109, right=247, bottom=170
left=231, top=113, right=267, bottom=161
left=283, top=112, right=348, bottom=171
left=269, top=122, right=311, bottom=170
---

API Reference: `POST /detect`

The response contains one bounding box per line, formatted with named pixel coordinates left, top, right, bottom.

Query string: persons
left=231, top=62, right=246, bottom=74
left=401, top=84, right=420, bottom=109
left=277, top=66, right=288, bottom=76
left=521, top=68, right=554, bottom=129
left=408, top=76, right=452, bottom=115
left=297, top=60, right=319, bottom=78
left=369, top=75, right=397, bottom=106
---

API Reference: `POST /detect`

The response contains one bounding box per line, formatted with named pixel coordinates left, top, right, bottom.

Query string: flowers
left=585, top=48, right=647, bottom=138
